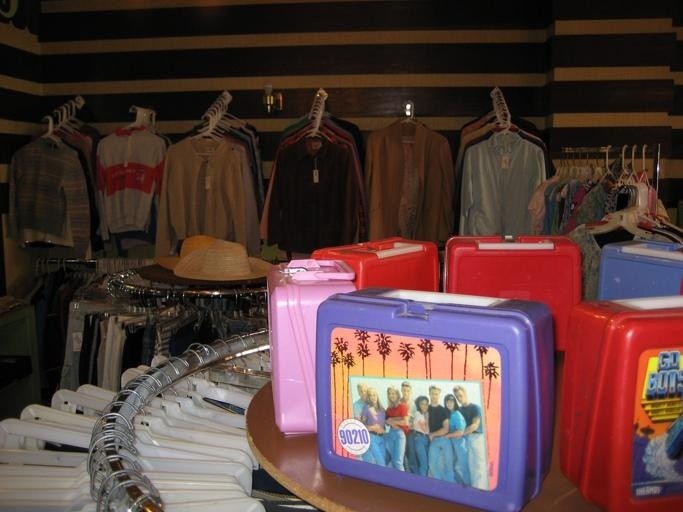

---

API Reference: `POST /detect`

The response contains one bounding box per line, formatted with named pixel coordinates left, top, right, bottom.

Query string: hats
left=156, top=234, right=209, bottom=270
left=174, top=238, right=273, bottom=281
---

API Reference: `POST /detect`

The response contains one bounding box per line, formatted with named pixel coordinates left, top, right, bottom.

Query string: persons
left=362, top=387, right=388, bottom=467
left=387, top=382, right=419, bottom=474
left=444, top=395, right=472, bottom=486
left=453, top=386, right=487, bottom=491
left=428, top=385, right=456, bottom=483
left=352, top=382, right=392, bottom=467
left=413, top=396, right=430, bottom=477
left=385, top=386, right=409, bottom=471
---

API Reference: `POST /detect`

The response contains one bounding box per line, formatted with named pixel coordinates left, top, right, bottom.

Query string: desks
left=243, top=372, right=615, bottom=512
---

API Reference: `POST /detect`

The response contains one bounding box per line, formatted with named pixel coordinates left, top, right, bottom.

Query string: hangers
left=488, top=86, right=524, bottom=143
left=295, top=87, right=335, bottom=146
left=70, top=268, right=268, bottom=334
left=588, top=182, right=683, bottom=243
left=39, top=99, right=80, bottom=146
left=398, top=100, right=417, bottom=127
left=554, top=143, right=652, bottom=204
left=190, top=90, right=243, bottom=156
left=33, top=254, right=156, bottom=276
left=0, top=326, right=271, bottom=512
left=122, top=105, right=157, bottom=132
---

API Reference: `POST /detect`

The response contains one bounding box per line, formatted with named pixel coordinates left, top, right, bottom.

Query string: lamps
left=261, top=82, right=283, bottom=113
